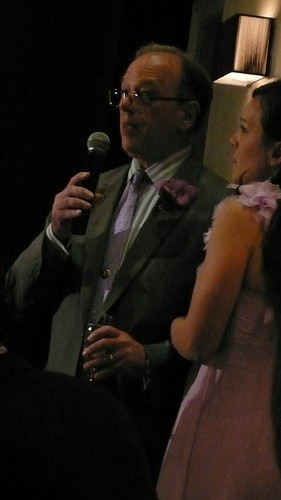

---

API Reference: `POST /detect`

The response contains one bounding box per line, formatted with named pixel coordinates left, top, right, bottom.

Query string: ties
left=89, top=170, right=148, bottom=317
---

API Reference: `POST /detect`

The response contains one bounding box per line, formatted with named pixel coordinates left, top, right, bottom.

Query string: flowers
left=154, top=179, right=198, bottom=210
left=238, top=179, right=281, bottom=218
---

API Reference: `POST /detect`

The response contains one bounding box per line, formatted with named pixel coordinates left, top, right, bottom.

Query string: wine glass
left=83, top=311, right=113, bottom=385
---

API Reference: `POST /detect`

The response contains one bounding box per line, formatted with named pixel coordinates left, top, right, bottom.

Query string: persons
left=1, top=45, right=237, bottom=386
left=1, top=343, right=156, bottom=500
left=155, top=79, right=281, bottom=500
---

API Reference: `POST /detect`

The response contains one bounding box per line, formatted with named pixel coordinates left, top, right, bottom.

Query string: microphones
left=71, top=132, right=110, bottom=235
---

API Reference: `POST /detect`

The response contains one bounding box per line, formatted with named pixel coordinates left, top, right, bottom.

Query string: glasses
left=108, top=88, right=192, bottom=107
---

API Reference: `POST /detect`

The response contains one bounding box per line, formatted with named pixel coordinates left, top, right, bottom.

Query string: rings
left=109, top=354, right=115, bottom=362
